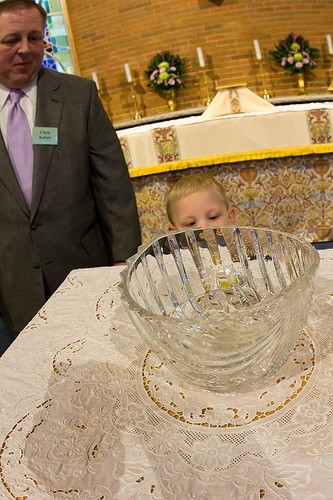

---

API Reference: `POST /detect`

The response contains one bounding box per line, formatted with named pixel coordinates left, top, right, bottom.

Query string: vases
left=164, top=90, right=177, bottom=112
left=296, top=73, right=306, bottom=95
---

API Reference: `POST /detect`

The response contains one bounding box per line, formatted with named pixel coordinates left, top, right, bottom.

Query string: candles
left=326, top=34, right=333, bottom=54
left=92, top=72, right=99, bottom=90
left=124, top=63, right=132, bottom=82
left=196, top=47, right=205, bottom=67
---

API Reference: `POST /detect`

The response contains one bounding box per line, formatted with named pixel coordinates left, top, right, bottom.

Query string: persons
left=0, top=0, right=142, bottom=340
left=166, top=174, right=241, bottom=247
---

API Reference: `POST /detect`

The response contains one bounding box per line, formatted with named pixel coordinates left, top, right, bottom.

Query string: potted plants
left=253, top=39, right=262, bottom=60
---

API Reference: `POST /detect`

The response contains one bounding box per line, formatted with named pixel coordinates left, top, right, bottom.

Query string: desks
left=0, top=250, right=333, bottom=500
left=115, top=101, right=333, bottom=246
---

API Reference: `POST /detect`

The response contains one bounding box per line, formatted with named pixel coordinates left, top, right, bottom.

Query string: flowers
left=143, top=50, right=188, bottom=96
left=268, top=31, right=320, bottom=77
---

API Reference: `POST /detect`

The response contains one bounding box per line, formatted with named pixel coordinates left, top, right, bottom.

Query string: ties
left=8, top=89, right=33, bottom=210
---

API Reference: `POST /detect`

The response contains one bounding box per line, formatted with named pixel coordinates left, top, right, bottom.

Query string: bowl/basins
left=118, top=227, right=320, bottom=393
